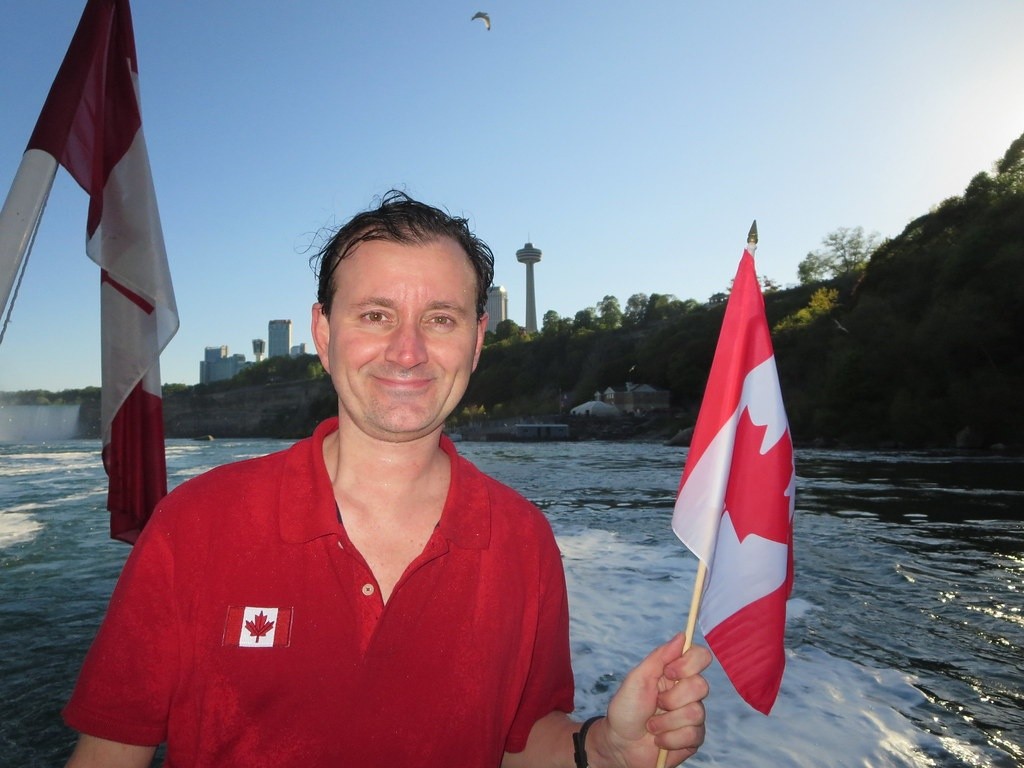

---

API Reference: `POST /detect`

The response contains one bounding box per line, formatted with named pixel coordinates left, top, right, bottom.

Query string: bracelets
left=572, top=714, right=605, bottom=767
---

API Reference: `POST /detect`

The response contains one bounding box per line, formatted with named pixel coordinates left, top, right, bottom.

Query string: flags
left=671, top=248, right=796, bottom=716
left=27, top=0, right=180, bottom=549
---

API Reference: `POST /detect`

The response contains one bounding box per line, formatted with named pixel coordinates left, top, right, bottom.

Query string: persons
left=60, top=189, right=714, bottom=768
